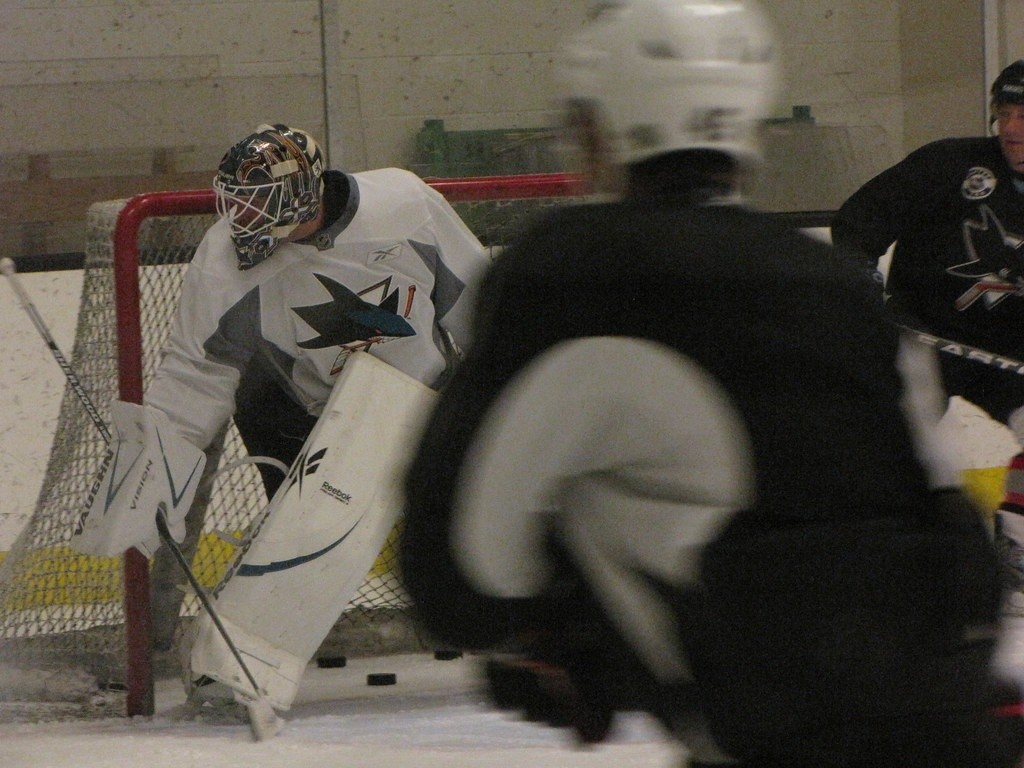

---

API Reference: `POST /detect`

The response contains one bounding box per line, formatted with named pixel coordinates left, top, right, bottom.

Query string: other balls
left=366, top=672, right=397, bottom=686
left=317, top=655, right=347, bottom=669
left=434, top=650, right=463, bottom=661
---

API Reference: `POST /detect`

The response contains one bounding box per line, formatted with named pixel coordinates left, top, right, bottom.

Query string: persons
left=68, top=122, right=496, bottom=715
left=395, top=1, right=1001, bottom=768
left=831, top=59, right=1024, bottom=618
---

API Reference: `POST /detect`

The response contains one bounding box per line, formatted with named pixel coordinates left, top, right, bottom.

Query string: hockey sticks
left=881, top=318, right=1024, bottom=377
left=0, top=255, right=285, bottom=742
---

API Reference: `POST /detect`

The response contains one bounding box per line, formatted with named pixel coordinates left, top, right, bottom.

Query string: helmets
left=548, top=0, right=777, bottom=172
left=212, top=123, right=326, bottom=270
left=989, top=59, right=1024, bottom=137
